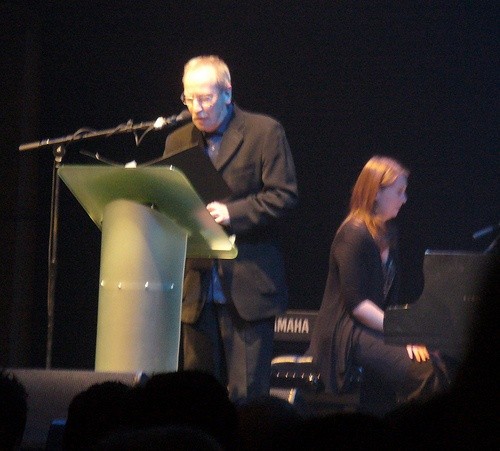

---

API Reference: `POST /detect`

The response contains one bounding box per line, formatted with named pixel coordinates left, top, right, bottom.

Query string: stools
left=267, top=360, right=366, bottom=388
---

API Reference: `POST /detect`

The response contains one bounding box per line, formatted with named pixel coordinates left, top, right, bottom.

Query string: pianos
left=382, top=226, right=500, bottom=379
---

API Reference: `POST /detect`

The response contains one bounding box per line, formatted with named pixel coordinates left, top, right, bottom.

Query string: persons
left=160, top=55, right=300, bottom=405
left=315, top=154, right=455, bottom=406
left=0, top=368, right=500, bottom=451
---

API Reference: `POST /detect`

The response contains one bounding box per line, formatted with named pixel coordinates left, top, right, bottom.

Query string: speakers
left=6, top=367, right=149, bottom=438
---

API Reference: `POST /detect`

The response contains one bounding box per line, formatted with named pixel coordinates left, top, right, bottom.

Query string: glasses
left=180, top=93, right=214, bottom=106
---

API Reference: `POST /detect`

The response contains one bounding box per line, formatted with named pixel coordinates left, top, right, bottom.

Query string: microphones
left=152, top=110, right=192, bottom=130
